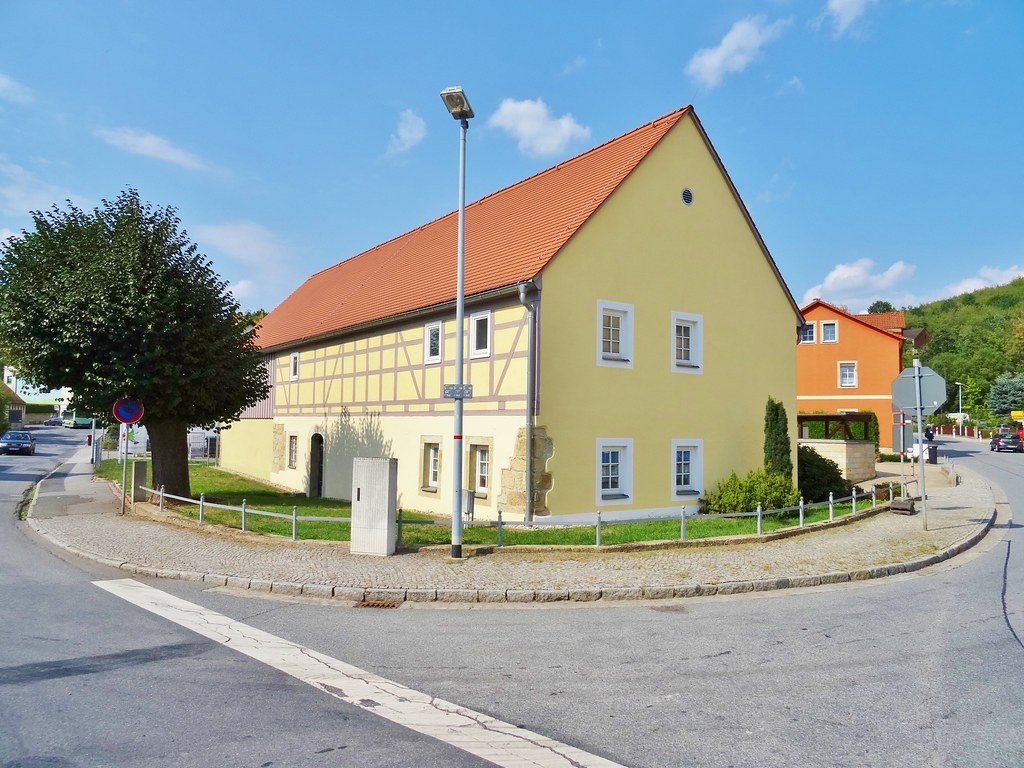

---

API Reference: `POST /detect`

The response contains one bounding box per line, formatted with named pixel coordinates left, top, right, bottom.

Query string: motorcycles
left=924, top=428, right=935, bottom=441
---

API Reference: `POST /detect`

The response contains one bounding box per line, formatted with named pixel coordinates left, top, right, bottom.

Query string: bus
left=60, top=410, right=93, bottom=429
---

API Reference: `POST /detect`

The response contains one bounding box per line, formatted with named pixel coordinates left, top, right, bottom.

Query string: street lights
left=54, top=398, right=64, bottom=416
left=955, top=382, right=962, bottom=436
left=436, top=82, right=476, bottom=561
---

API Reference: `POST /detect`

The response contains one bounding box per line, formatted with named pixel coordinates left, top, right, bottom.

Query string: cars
left=44, top=417, right=63, bottom=426
left=0, top=431, right=37, bottom=456
left=989, top=432, right=1023, bottom=453
